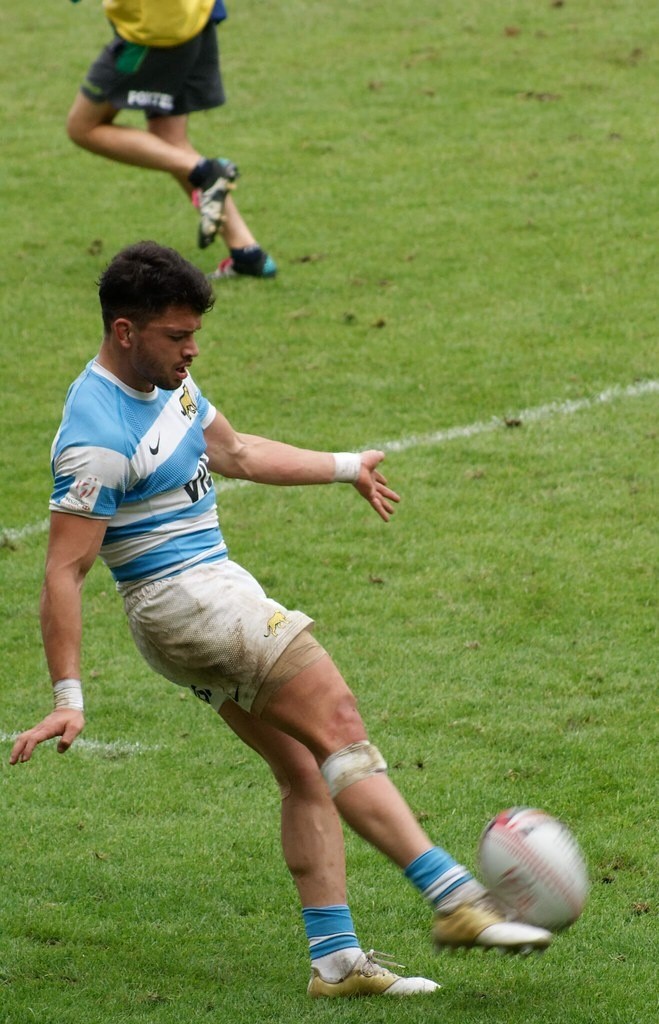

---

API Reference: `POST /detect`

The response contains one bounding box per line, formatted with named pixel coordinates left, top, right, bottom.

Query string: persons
left=8, top=240, right=554, bottom=997
left=66, top=0, right=278, bottom=279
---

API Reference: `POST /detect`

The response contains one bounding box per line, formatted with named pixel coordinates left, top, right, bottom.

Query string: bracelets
left=332, top=452, right=361, bottom=485
left=53, top=678, right=84, bottom=711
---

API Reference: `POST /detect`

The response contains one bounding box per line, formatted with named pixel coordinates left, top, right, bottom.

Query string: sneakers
left=434, top=891, right=552, bottom=957
left=305, top=950, right=442, bottom=1002
left=190, top=156, right=240, bottom=249
left=206, top=246, right=279, bottom=282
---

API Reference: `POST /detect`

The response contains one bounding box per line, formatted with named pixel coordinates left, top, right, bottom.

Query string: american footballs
left=475, top=803, right=593, bottom=932
